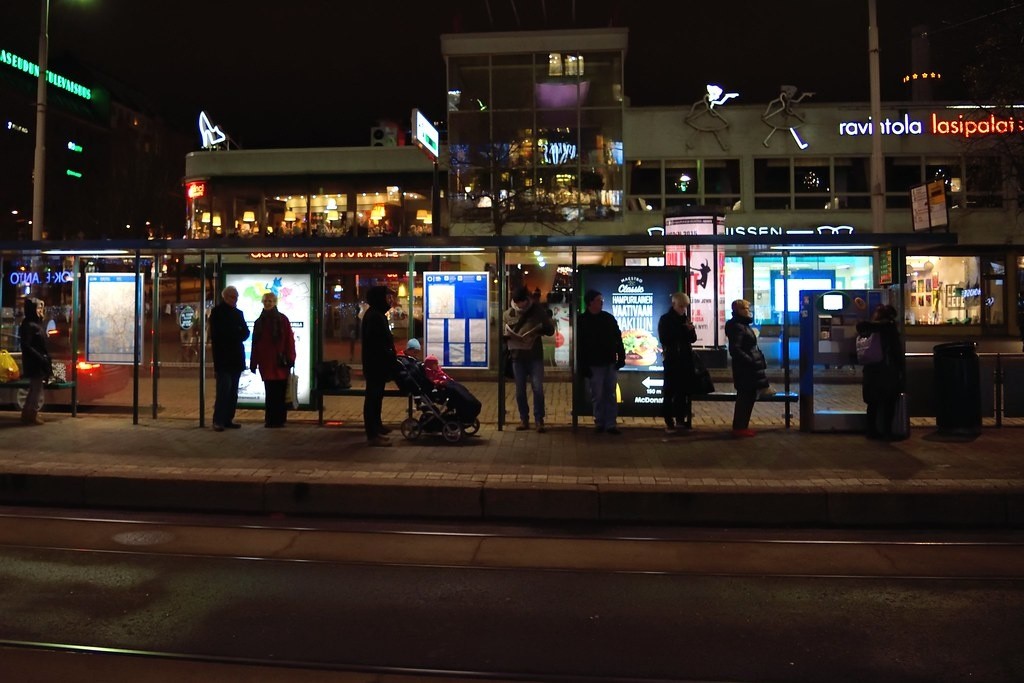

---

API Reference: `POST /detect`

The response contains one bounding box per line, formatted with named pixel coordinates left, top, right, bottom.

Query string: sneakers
left=368, top=437, right=392, bottom=447
left=378, top=432, right=390, bottom=440
left=516, top=420, right=531, bottom=430
left=607, top=428, right=621, bottom=435
left=536, top=420, right=544, bottom=433
left=595, top=425, right=603, bottom=434
left=212, top=422, right=225, bottom=432
left=224, top=419, right=241, bottom=429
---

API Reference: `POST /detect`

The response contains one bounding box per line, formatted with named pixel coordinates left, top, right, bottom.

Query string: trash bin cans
left=934, top=341, right=982, bottom=440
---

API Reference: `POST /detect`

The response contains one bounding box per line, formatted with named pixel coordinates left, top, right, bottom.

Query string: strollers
left=396, top=355, right=481, bottom=443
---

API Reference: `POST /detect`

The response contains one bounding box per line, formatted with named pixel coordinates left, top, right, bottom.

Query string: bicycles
left=175, top=329, right=200, bottom=371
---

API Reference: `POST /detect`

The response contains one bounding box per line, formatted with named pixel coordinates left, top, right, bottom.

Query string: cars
left=0, top=317, right=132, bottom=412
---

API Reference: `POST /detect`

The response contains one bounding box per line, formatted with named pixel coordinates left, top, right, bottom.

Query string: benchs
left=321, top=387, right=410, bottom=397
left=0, top=375, right=77, bottom=390
left=691, top=391, right=798, bottom=401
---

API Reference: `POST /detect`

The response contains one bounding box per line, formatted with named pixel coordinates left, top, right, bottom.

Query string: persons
left=363, top=216, right=370, bottom=236
left=409, top=225, right=422, bottom=236
left=284, top=222, right=292, bottom=234
left=234, top=223, right=242, bottom=236
left=209, top=286, right=250, bottom=432
left=503, top=286, right=555, bottom=432
left=275, top=221, right=283, bottom=234
left=19, top=297, right=54, bottom=424
left=658, top=291, right=697, bottom=432
left=856, top=302, right=907, bottom=442
left=370, top=219, right=377, bottom=233
left=250, top=292, right=296, bottom=428
left=424, top=353, right=453, bottom=386
left=292, top=218, right=303, bottom=235
left=725, top=299, right=770, bottom=437
left=323, top=219, right=331, bottom=235
left=396, top=339, right=427, bottom=410
left=361, top=285, right=402, bottom=446
left=690, top=258, right=709, bottom=289
left=577, top=288, right=626, bottom=435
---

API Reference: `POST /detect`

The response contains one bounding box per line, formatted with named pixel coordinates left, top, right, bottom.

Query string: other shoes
left=665, top=416, right=674, bottom=429
left=732, top=427, right=756, bottom=438
left=881, top=434, right=898, bottom=441
left=23, top=415, right=42, bottom=424
left=675, top=421, right=688, bottom=428
left=867, top=431, right=880, bottom=439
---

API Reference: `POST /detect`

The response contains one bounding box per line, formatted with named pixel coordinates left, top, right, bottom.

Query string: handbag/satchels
left=278, top=363, right=299, bottom=414
left=48, top=362, right=67, bottom=384
left=855, top=333, right=884, bottom=368
left=692, top=352, right=714, bottom=393
left=752, top=372, right=769, bottom=389
left=0, top=350, right=20, bottom=383
left=503, top=348, right=514, bottom=379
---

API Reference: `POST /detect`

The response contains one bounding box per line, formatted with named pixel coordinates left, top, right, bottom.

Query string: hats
left=425, top=354, right=438, bottom=367
left=512, top=287, right=527, bottom=302
left=584, top=290, right=602, bottom=305
left=407, top=337, right=423, bottom=350
